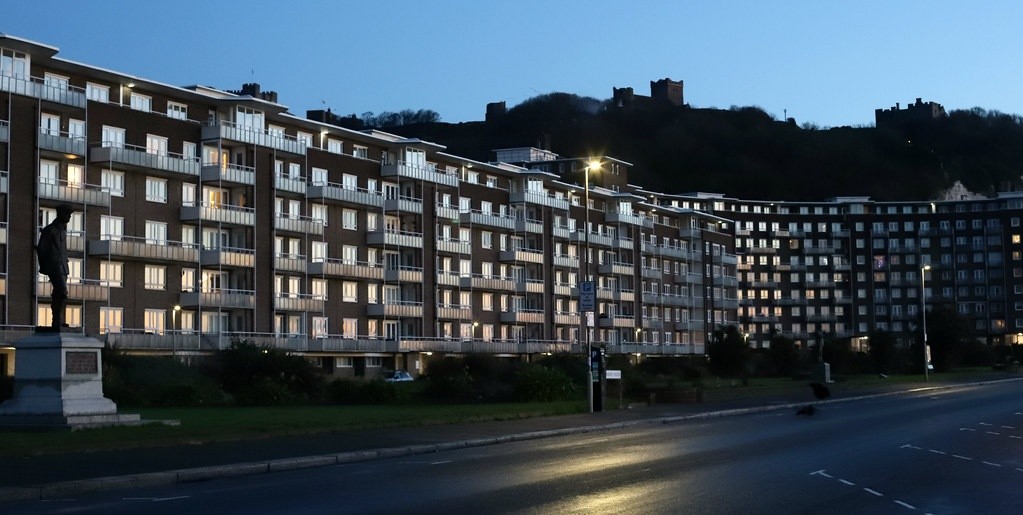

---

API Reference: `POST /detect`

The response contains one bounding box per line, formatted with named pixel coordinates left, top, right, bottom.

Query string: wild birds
left=808, top=382, right=832, bottom=401
left=795, top=405, right=816, bottom=417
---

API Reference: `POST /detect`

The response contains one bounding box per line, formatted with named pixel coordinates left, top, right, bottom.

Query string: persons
left=815, top=324, right=826, bottom=363
left=37, top=205, right=74, bottom=327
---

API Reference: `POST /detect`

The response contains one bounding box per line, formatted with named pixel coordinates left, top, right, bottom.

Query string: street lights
left=584, top=160, right=600, bottom=412
left=922, top=265, right=930, bottom=382
